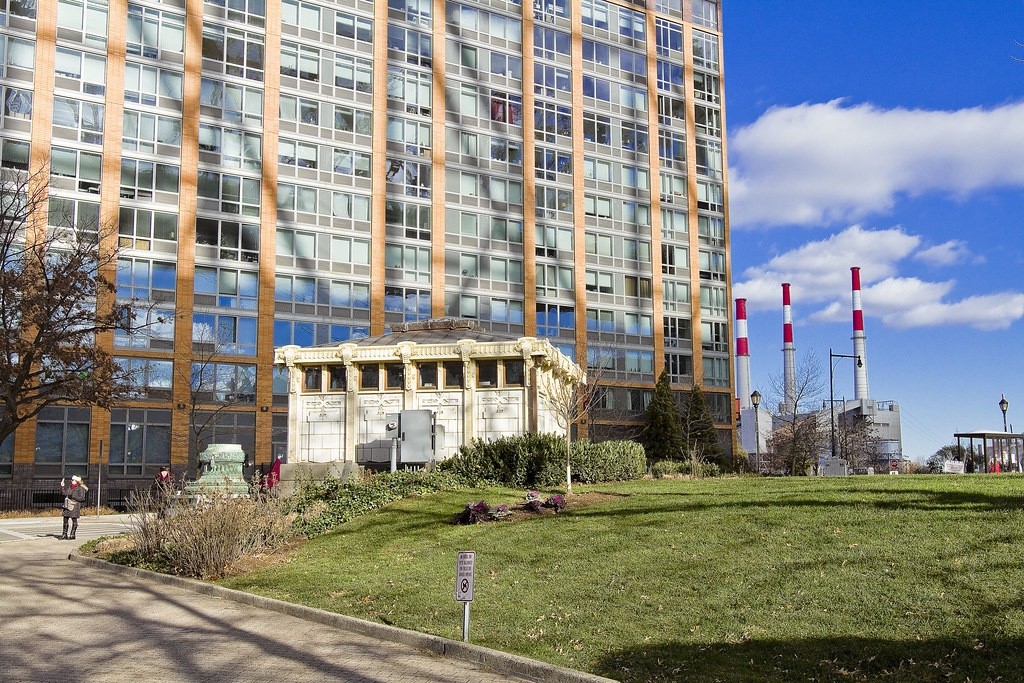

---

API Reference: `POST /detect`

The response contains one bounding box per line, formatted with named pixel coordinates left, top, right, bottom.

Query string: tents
left=253, top=458, right=283, bottom=488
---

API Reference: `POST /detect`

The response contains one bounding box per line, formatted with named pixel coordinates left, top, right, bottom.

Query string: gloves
left=68, top=495, right=72, bottom=499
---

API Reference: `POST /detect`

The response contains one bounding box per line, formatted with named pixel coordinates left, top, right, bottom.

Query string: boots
left=59, top=524, right=77, bottom=540
left=158, top=508, right=166, bottom=519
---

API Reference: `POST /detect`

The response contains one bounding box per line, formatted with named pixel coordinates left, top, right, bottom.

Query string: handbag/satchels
left=62, top=496, right=77, bottom=511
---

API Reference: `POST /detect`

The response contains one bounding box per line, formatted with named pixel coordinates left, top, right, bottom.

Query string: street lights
left=999, top=393, right=1008, bottom=432
left=830, top=348, right=862, bottom=456
left=750, top=390, right=761, bottom=474
left=822, top=396, right=848, bottom=461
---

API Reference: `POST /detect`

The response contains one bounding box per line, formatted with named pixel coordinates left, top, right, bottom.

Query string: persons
left=59, top=474, right=88, bottom=540
left=150, top=466, right=174, bottom=520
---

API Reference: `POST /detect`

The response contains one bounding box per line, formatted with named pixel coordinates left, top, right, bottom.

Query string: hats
left=160, top=466, right=168, bottom=472
left=72, top=474, right=81, bottom=481
